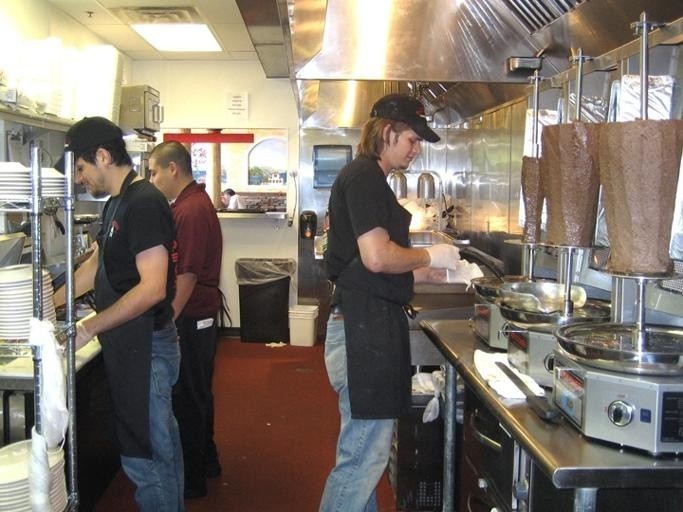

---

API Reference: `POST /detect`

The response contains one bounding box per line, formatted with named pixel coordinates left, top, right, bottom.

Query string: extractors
left=293, top=1, right=683, bottom=88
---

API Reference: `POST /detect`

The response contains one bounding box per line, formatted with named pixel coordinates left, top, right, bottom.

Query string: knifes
left=494, top=359, right=559, bottom=421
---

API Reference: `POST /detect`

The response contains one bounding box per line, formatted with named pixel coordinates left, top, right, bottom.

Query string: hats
left=54, top=117, right=122, bottom=175
left=371, top=94, right=441, bottom=143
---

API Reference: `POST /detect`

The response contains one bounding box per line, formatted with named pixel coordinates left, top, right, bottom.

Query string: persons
left=215, top=187, right=250, bottom=209
left=41, top=115, right=187, bottom=511
left=311, top=94, right=479, bottom=511
left=140, top=141, right=226, bottom=501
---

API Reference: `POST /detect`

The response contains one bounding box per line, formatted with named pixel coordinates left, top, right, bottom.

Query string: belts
left=445, top=260, right=485, bottom=289
left=425, top=244, right=460, bottom=269
left=330, top=303, right=339, bottom=313
left=152, top=320, right=174, bottom=330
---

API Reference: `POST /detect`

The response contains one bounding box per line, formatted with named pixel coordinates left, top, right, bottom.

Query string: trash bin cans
left=237, top=258, right=293, bottom=343
left=396, top=363, right=457, bottom=512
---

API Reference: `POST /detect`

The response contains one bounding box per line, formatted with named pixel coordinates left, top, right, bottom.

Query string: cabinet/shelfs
left=0, top=147, right=81, bottom=511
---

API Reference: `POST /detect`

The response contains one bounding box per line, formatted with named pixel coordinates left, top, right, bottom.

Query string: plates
left=0, top=262, right=58, bottom=340
left=0, top=160, right=68, bottom=200
left=0, top=438, right=69, bottom=512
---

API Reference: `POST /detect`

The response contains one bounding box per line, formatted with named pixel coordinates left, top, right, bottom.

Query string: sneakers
left=185, top=486, right=208, bottom=498
left=207, top=467, right=221, bottom=477
